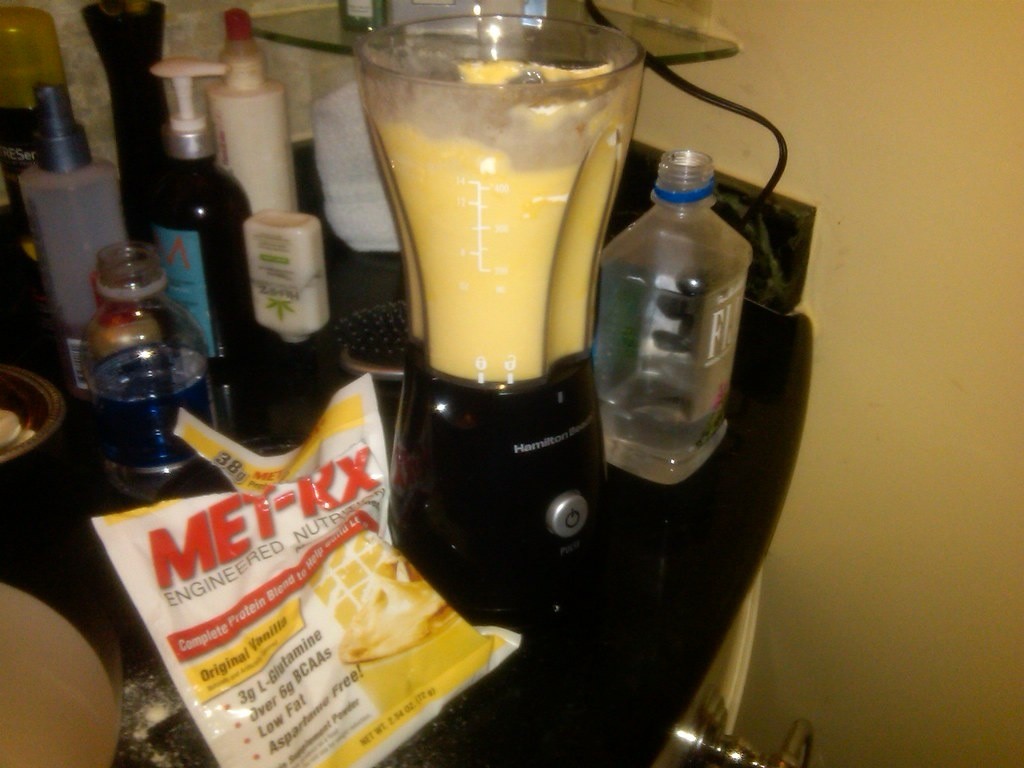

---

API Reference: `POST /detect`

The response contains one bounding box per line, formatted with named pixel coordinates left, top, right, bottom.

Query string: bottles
left=592, top=149, right=754, bottom=485
left=78, top=240, right=222, bottom=510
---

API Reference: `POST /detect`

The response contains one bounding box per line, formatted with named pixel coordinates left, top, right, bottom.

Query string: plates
left=0, top=582, right=117, bottom=768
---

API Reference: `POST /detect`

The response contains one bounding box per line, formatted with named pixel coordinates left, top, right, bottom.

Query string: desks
left=0, top=139, right=818, bottom=768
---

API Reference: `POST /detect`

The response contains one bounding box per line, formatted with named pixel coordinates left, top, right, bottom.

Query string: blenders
left=349, top=14, right=644, bottom=631
